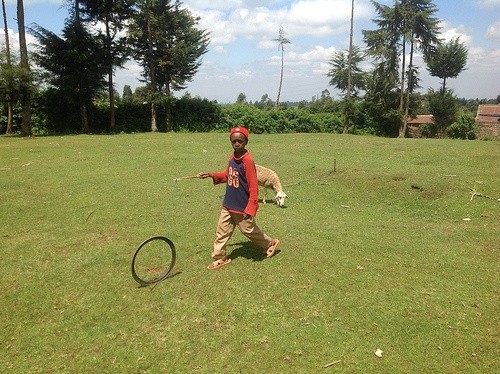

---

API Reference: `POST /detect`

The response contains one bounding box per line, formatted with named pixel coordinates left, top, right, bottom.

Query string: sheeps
left=255, top=164, right=288, bottom=207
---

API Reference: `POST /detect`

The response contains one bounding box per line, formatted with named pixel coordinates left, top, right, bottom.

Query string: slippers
left=207, top=258, right=232, bottom=270
left=262, top=239, right=280, bottom=258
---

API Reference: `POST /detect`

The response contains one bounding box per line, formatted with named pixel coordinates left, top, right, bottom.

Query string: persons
left=196, top=127, right=280, bottom=271
